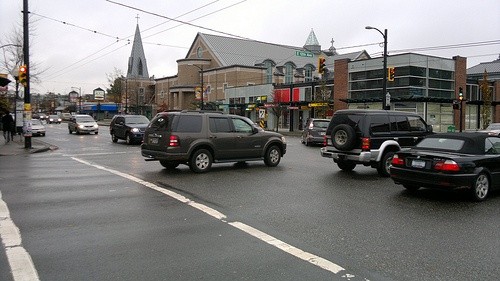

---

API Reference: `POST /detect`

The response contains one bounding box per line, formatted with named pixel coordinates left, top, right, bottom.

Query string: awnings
left=0, top=77, right=12, bottom=88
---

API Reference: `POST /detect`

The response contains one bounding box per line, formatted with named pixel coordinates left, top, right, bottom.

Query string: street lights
left=365, top=26, right=389, bottom=106
left=458, top=90, right=463, bottom=100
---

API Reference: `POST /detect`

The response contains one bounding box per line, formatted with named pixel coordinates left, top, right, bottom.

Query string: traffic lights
left=389, top=66, right=395, bottom=82
left=318, top=57, right=326, bottom=73
left=18, top=65, right=27, bottom=85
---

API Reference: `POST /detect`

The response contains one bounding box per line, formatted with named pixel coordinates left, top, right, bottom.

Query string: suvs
left=142, top=110, right=286, bottom=173
left=320, top=109, right=433, bottom=172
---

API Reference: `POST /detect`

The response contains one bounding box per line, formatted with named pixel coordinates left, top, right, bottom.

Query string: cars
left=68, top=113, right=97, bottom=135
left=32, top=113, right=47, bottom=121
left=385, top=131, right=500, bottom=202
left=110, top=113, right=151, bottom=142
left=47, top=115, right=62, bottom=123
left=300, top=118, right=331, bottom=147
left=485, top=123, right=500, bottom=131
left=31, top=119, right=46, bottom=137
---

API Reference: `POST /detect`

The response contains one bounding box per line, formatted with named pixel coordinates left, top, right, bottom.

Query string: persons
left=0, top=110, right=24, bottom=143
